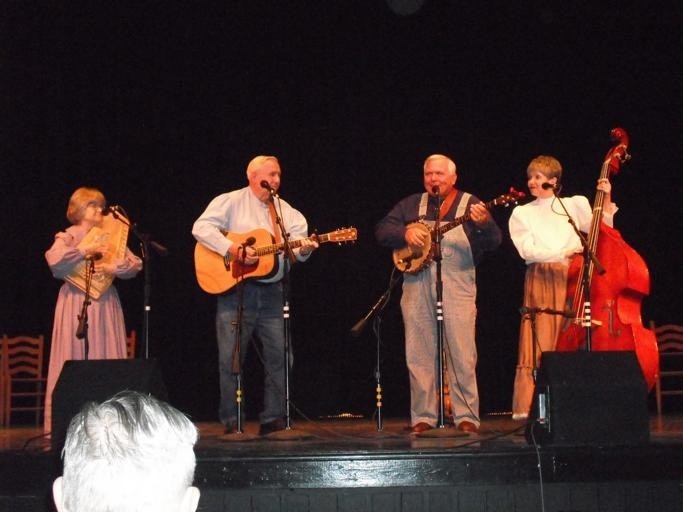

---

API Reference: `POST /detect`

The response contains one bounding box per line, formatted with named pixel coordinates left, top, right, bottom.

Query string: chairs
left=124, top=330, right=134, bottom=358
left=0, top=336, right=6, bottom=425
left=649, top=319, right=682, bottom=416
left=4, top=334, right=47, bottom=428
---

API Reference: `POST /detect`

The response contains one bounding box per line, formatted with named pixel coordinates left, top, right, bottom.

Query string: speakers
left=526, top=351, right=650, bottom=448
left=51, top=359, right=171, bottom=452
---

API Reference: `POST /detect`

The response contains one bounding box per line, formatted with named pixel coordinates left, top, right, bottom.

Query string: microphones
left=432, top=186, right=441, bottom=197
left=101, top=205, right=119, bottom=215
left=542, top=182, right=558, bottom=190
left=259, top=180, right=274, bottom=194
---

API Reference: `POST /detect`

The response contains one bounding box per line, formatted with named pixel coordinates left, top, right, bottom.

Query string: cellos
left=558, top=128, right=659, bottom=393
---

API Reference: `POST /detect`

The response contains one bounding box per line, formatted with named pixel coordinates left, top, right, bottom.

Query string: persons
left=51, top=389, right=201, bottom=512
left=191, top=157, right=319, bottom=434
left=510, top=154, right=620, bottom=421
left=44, top=184, right=143, bottom=439
left=374, top=154, right=501, bottom=434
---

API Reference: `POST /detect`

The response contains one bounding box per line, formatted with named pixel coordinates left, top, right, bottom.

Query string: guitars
left=193, top=226, right=357, bottom=296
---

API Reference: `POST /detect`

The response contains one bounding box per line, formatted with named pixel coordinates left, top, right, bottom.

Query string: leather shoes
left=413, top=422, right=431, bottom=432
left=224, top=422, right=237, bottom=433
left=259, top=424, right=275, bottom=434
left=458, top=421, right=474, bottom=431
